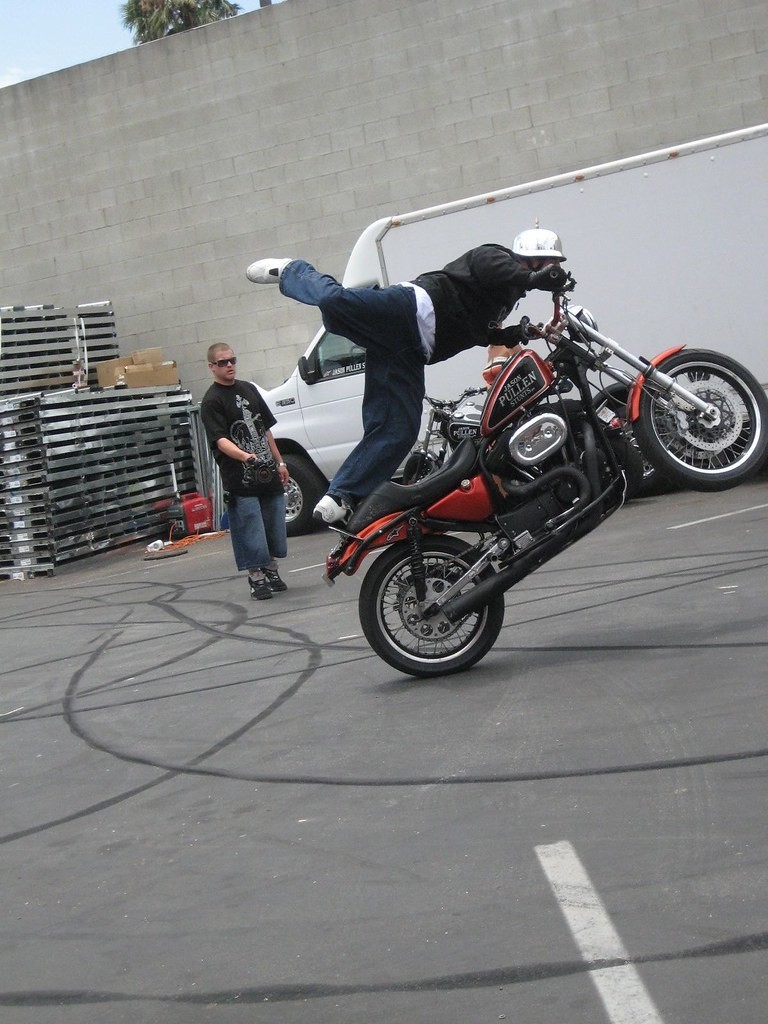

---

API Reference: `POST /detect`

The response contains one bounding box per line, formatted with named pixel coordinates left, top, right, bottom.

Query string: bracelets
left=276, top=463, right=287, bottom=467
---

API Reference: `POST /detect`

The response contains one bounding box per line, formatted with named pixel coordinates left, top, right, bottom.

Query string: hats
left=511, top=229, right=567, bottom=262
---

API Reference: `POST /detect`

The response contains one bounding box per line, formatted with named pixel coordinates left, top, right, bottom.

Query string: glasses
left=212, top=357, right=237, bottom=367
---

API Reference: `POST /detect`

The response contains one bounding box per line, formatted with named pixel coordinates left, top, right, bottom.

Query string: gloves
left=502, top=324, right=529, bottom=349
left=525, top=264, right=567, bottom=291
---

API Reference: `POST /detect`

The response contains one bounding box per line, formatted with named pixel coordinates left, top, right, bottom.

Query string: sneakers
left=245, top=258, right=294, bottom=284
left=312, top=494, right=351, bottom=525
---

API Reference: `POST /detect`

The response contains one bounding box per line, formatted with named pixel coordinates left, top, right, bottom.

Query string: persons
left=200, top=342, right=291, bottom=601
left=246, top=227, right=568, bottom=524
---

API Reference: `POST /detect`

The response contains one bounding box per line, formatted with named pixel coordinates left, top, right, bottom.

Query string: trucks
left=250, top=124, right=767, bottom=536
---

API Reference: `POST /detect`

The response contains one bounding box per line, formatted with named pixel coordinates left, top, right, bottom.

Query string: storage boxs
left=124, top=360, right=179, bottom=388
left=95, top=356, right=134, bottom=386
left=133, top=346, right=164, bottom=364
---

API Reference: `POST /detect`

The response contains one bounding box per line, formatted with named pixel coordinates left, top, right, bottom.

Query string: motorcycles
left=321, top=267, right=768, bottom=678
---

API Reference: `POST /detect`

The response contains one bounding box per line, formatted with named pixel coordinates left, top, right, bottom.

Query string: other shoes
left=247, top=567, right=273, bottom=600
left=259, top=559, right=288, bottom=592
